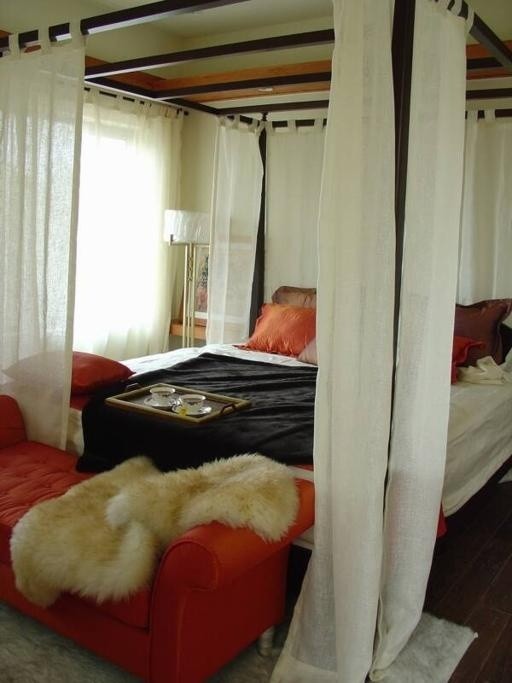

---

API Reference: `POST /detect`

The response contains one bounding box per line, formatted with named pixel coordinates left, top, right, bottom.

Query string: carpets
left=1, top=610, right=479, bottom=682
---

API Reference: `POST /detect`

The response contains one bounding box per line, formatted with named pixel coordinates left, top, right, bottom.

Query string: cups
left=179, top=394, right=206, bottom=412
left=149, top=386, right=176, bottom=405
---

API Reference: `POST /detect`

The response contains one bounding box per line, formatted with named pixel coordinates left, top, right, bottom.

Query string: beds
left=0, top=0, right=511, bottom=682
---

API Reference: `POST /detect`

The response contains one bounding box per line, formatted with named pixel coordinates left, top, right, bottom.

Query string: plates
left=172, top=403, right=212, bottom=415
left=143, top=396, right=177, bottom=411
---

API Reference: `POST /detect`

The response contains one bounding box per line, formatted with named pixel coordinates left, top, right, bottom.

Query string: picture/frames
left=177, top=244, right=209, bottom=326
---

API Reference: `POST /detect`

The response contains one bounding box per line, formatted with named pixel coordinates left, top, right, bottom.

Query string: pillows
left=235, top=286, right=512, bottom=385
left=3, top=349, right=137, bottom=393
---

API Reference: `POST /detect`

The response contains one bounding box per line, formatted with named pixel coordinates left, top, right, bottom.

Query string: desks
left=170, top=319, right=206, bottom=340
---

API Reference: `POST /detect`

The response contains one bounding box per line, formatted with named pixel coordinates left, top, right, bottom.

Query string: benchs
left=1, top=395, right=315, bottom=680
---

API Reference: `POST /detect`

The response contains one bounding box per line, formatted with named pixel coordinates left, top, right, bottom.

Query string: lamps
left=164, top=209, right=210, bottom=349
